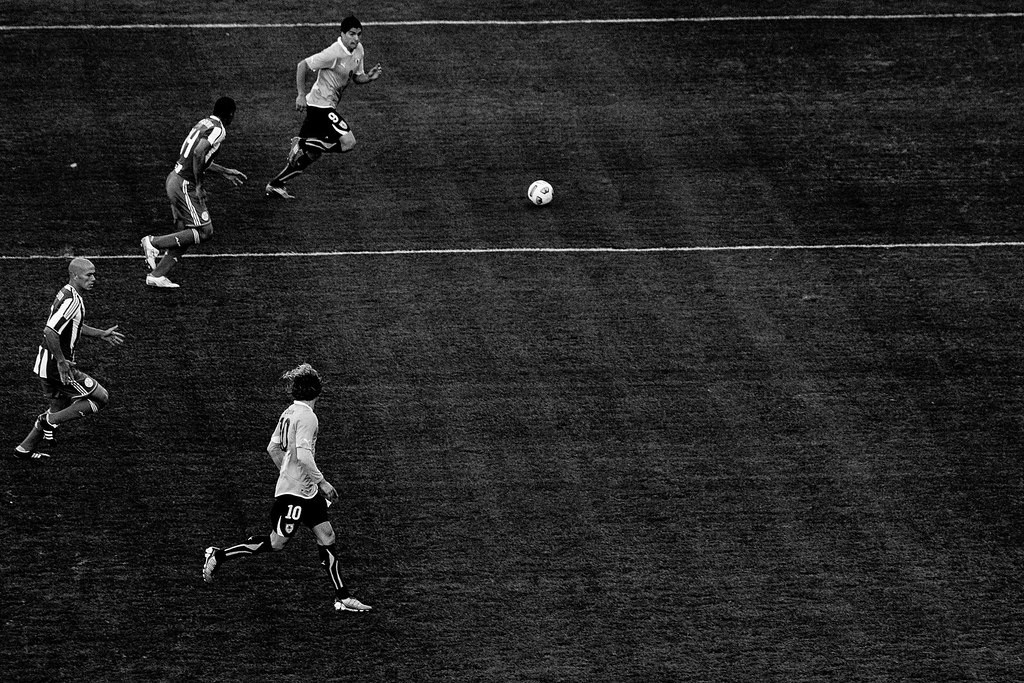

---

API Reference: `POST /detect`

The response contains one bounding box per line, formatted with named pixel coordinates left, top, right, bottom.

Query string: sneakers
left=37, top=413, right=58, bottom=446
left=334, top=596, right=372, bottom=611
left=202, top=547, right=219, bottom=584
left=287, top=134, right=302, bottom=166
left=144, top=271, right=181, bottom=289
left=139, top=235, right=159, bottom=271
left=264, top=182, right=296, bottom=201
left=13, top=446, right=50, bottom=461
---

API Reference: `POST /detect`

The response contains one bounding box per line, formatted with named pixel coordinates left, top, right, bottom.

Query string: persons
left=264, top=17, right=382, bottom=200
left=13, top=258, right=126, bottom=460
left=140, top=97, right=248, bottom=289
left=202, top=362, right=375, bottom=614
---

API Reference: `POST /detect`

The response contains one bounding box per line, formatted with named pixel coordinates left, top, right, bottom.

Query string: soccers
left=525, top=179, right=554, bottom=203
